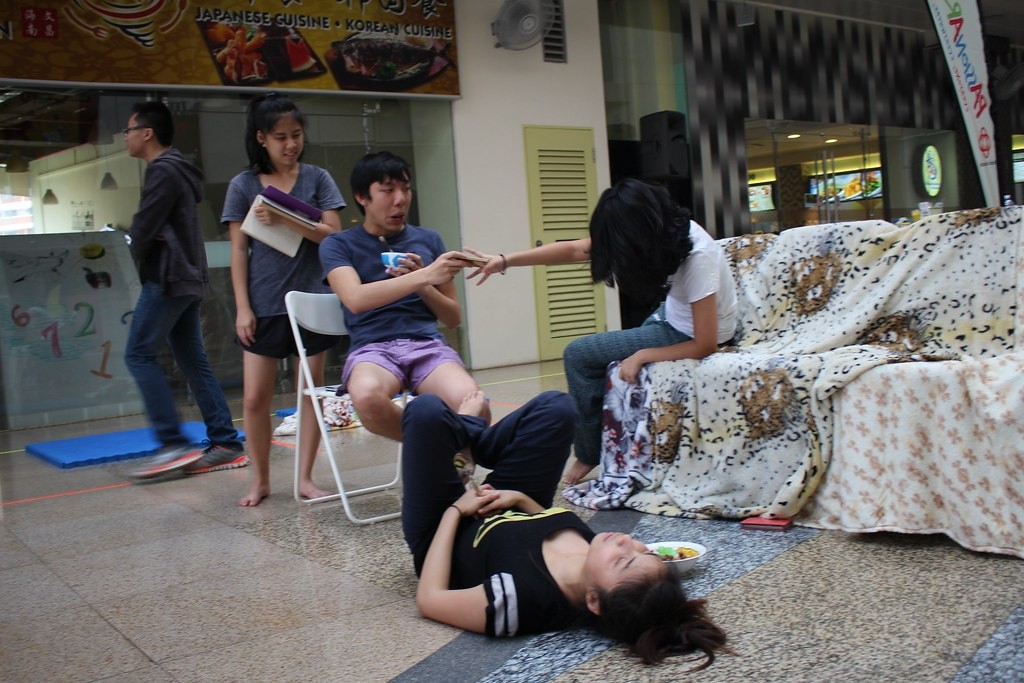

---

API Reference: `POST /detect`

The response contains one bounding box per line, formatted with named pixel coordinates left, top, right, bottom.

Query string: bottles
left=893, top=201, right=944, bottom=228
left=1004, top=195, right=1014, bottom=206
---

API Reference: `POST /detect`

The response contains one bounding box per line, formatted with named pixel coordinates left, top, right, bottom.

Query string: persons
left=398, top=392, right=735, bottom=676
left=221, top=90, right=347, bottom=506
left=316, top=152, right=477, bottom=445
left=121, top=101, right=250, bottom=480
left=460, top=180, right=739, bottom=487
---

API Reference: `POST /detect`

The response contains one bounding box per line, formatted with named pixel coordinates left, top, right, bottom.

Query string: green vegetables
left=374, top=60, right=397, bottom=80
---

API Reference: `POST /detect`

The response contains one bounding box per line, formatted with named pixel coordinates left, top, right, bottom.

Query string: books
left=239, top=183, right=325, bottom=258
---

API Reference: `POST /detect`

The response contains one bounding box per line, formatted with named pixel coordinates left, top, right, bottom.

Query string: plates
left=822, top=187, right=863, bottom=202
left=323, top=35, right=449, bottom=92
left=196, top=15, right=327, bottom=87
left=748, top=186, right=772, bottom=210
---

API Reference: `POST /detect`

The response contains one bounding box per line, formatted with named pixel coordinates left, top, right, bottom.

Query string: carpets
left=386, top=503, right=1024, bottom=683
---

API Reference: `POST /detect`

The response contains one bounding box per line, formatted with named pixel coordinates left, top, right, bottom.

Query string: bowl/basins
left=645, top=541, right=706, bottom=573
left=381, top=252, right=409, bottom=269
left=339, top=32, right=434, bottom=86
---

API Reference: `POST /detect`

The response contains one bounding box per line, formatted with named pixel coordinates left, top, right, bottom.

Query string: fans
left=491, top=0, right=555, bottom=51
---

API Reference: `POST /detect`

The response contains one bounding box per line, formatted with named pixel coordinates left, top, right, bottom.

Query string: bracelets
left=452, top=504, right=462, bottom=515
left=498, top=253, right=507, bottom=276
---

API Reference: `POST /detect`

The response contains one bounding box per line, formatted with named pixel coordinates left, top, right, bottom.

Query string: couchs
left=562, top=204, right=1024, bottom=560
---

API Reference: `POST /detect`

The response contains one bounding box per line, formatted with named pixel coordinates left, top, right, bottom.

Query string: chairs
left=285, top=291, right=417, bottom=526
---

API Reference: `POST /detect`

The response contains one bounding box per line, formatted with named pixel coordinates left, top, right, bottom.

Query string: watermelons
left=284, top=36, right=320, bottom=73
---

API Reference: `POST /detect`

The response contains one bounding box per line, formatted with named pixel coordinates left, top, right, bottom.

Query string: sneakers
left=128, top=443, right=204, bottom=477
left=184, top=439, right=250, bottom=474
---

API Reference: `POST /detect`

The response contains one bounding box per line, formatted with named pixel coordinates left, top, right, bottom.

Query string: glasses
left=122, top=125, right=156, bottom=135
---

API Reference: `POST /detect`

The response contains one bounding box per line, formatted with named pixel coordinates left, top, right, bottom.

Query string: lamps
left=4, top=89, right=118, bottom=204
